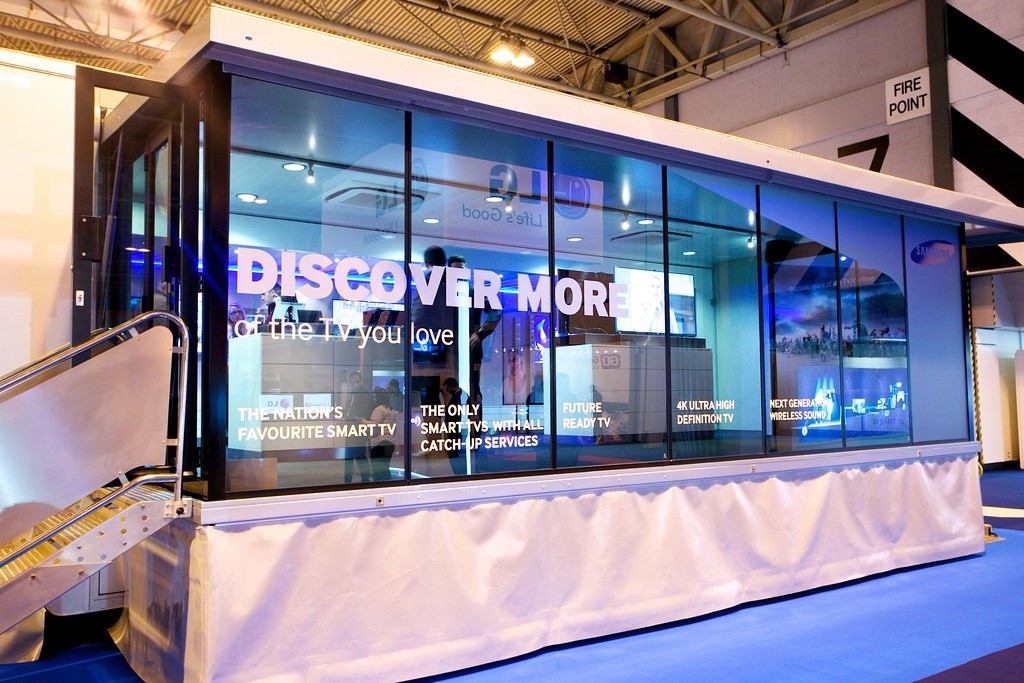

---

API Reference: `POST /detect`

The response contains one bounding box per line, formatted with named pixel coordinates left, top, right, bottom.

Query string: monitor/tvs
left=613, top=266, right=697, bottom=337
left=556, top=269, right=617, bottom=335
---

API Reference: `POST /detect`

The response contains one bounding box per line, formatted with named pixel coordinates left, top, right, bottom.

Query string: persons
left=260, top=282, right=281, bottom=324
left=133, top=275, right=172, bottom=329
left=415, top=245, right=504, bottom=457
left=228, top=303, right=247, bottom=338
left=441, top=372, right=473, bottom=476
left=341, top=371, right=405, bottom=484
left=504, top=347, right=528, bottom=404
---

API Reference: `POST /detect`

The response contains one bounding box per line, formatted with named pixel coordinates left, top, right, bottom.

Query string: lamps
left=492, top=34, right=514, bottom=63
left=514, top=41, right=536, bottom=68
